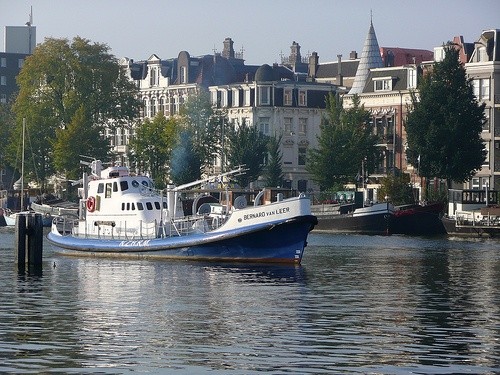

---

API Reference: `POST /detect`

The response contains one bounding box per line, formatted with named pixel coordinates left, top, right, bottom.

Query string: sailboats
left=0, top=115, right=80, bottom=227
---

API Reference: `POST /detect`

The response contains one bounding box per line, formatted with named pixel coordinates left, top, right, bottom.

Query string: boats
left=308, top=200, right=397, bottom=237
left=443, top=187, right=500, bottom=243
left=44, top=152, right=319, bottom=269
left=394, top=198, right=445, bottom=237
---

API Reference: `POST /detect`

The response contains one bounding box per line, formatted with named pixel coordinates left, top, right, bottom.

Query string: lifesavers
left=46, top=213, right=51, bottom=219
left=86, top=196, right=95, bottom=212
